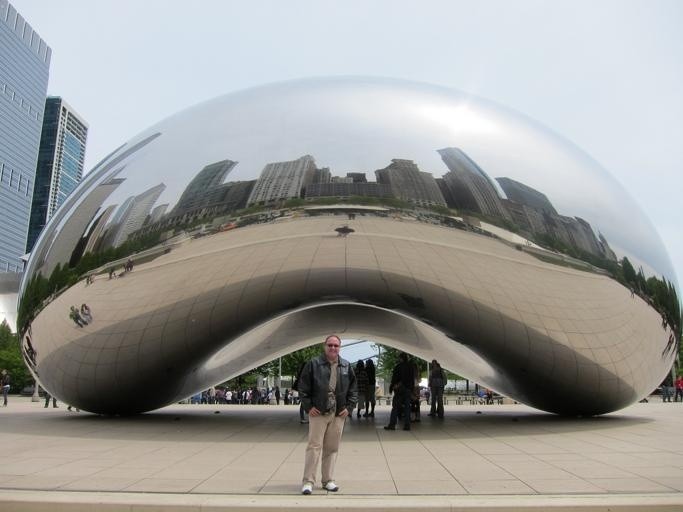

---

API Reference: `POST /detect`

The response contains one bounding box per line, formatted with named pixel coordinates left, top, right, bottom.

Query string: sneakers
left=323, top=482, right=337, bottom=491
left=300, top=419, right=309, bottom=422
left=301, top=482, right=313, bottom=494
left=356, top=412, right=374, bottom=418
left=428, top=411, right=444, bottom=415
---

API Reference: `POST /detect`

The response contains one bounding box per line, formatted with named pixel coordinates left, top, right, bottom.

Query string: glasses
left=327, top=344, right=339, bottom=348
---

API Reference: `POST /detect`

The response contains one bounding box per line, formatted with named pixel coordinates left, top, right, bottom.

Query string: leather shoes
left=384, top=425, right=410, bottom=431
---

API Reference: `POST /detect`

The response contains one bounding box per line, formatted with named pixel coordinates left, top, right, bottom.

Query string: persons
left=298, top=335, right=359, bottom=495
left=486, top=388, right=493, bottom=405
left=661, top=311, right=678, bottom=359
left=660, top=377, right=671, bottom=402
left=20, top=323, right=39, bottom=379
left=68, top=306, right=89, bottom=327
left=630, top=287, right=636, bottom=298
left=673, top=375, right=682, bottom=402
left=67, top=405, right=81, bottom=412
left=477, top=388, right=485, bottom=404
left=203, top=351, right=447, bottom=432
left=1, top=369, right=10, bottom=407
left=86, top=257, right=134, bottom=283
left=80, top=304, right=93, bottom=322
left=43, top=392, right=58, bottom=408
